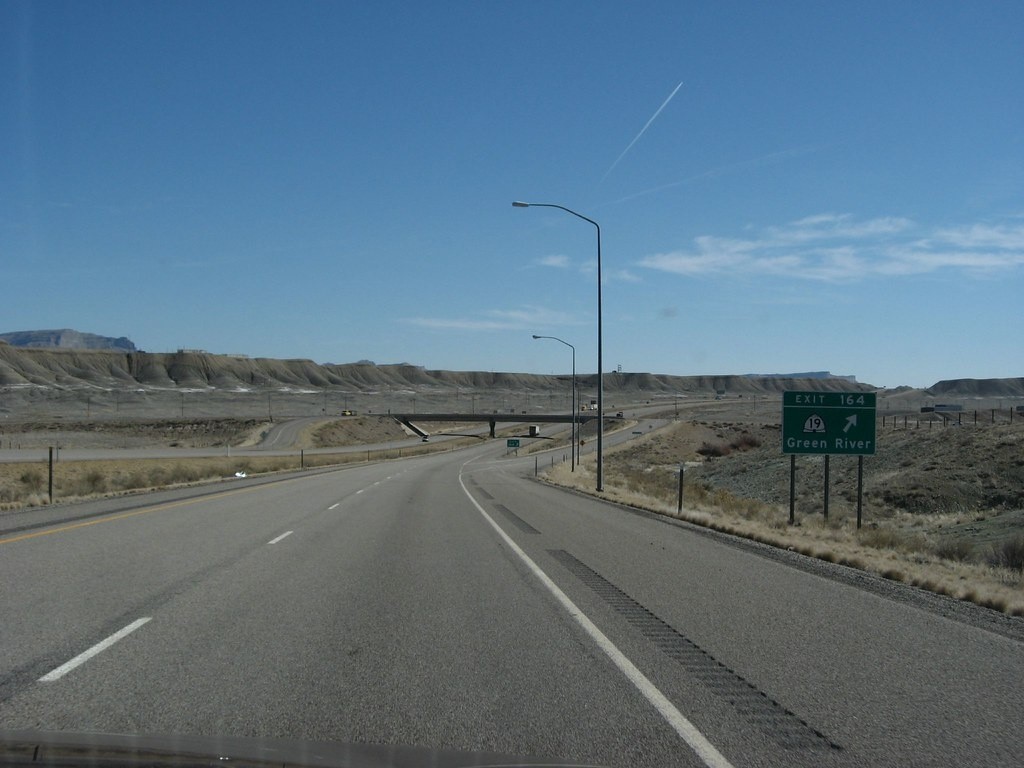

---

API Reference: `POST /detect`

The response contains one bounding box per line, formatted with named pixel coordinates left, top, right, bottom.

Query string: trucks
left=529, top=425, right=540, bottom=437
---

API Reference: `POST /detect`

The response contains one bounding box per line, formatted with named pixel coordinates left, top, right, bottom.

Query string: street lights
left=512, top=201, right=603, bottom=492
left=533, top=334, right=575, bottom=473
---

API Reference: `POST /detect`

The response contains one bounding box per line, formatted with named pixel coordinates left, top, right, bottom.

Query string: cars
left=341, top=410, right=352, bottom=416
left=422, top=435, right=429, bottom=442
